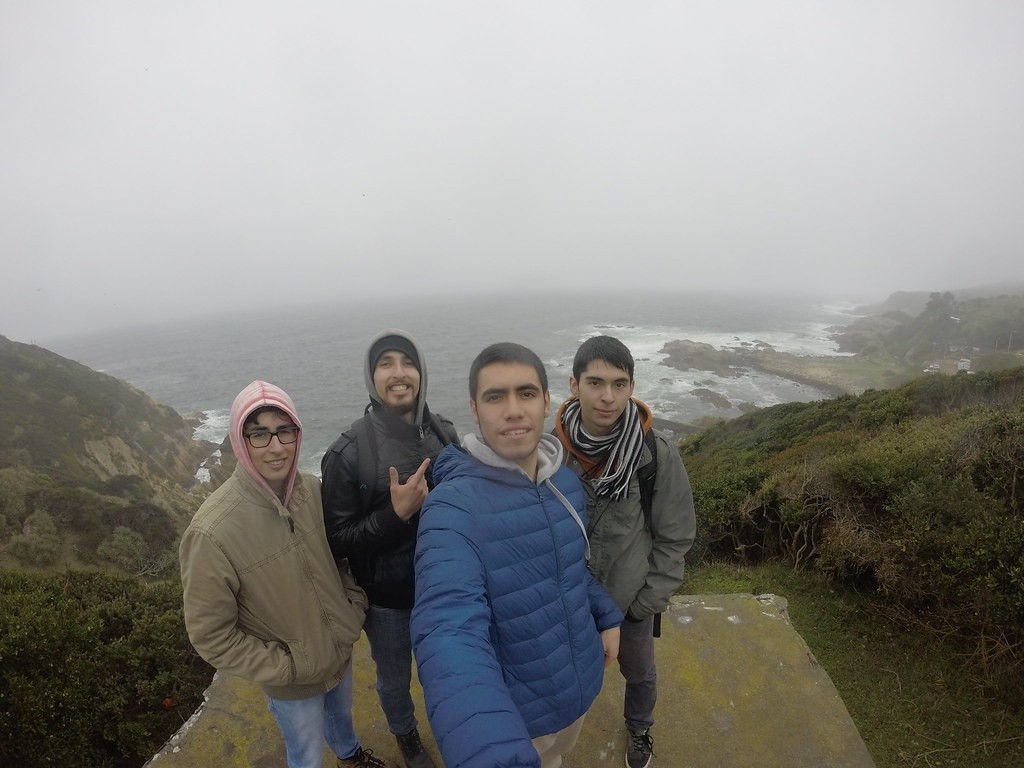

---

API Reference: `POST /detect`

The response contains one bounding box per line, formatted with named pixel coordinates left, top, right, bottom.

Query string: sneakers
left=625, top=726, right=653, bottom=768
left=338, top=747, right=399, bottom=767
left=391, top=730, right=435, bottom=768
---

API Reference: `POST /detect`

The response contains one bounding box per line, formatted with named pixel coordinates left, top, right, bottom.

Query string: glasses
left=242, top=425, right=300, bottom=449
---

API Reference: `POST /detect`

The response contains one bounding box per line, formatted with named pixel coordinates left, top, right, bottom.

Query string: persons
left=409, top=341, right=625, bottom=768
left=546, top=332, right=701, bottom=768
left=321, top=329, right=460, bottom=768
left=177, top=378, right=392, bottom=768
